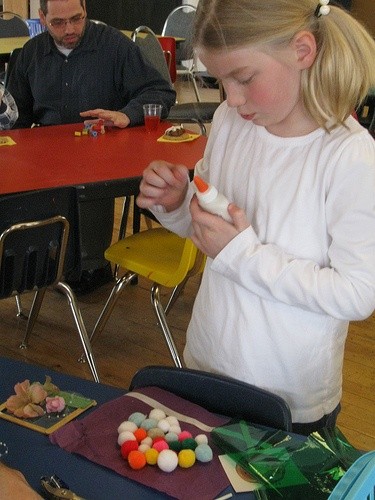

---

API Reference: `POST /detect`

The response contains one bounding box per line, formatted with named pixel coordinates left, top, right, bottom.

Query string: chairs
left=0, top=10, right=32, bottom=37
left=0, top=215, right=103, bottom=385
left=131, top=25, right=175, bottom=91
left=161, top=3, right=203, bottom=104
left=128, top=363, right=292, bottom=435
left=78, top=226, right=209, bottom=369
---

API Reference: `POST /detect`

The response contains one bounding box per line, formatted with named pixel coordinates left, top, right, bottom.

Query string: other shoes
left=56, top=263, right=112, bottom=296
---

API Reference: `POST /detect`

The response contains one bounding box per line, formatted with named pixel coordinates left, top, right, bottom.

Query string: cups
left=143, top=104, right=162, bottom=132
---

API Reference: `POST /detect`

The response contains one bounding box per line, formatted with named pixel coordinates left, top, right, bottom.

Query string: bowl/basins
left=27, top=19, right=48, bottom=39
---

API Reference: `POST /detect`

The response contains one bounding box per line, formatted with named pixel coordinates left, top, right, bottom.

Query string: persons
left=0, top=0, right=177, bottom=297
left=137, top=0, right=375, bottom=437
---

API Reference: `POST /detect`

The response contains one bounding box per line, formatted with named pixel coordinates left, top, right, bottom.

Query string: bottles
left=193, top=175, right=233, bottom=225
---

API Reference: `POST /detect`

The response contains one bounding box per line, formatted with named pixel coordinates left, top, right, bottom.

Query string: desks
left=0, top=120, right=208, bottom=286
left=0, top=358, right=370, bottom=500
left=0, top=29, right=186, bottom=82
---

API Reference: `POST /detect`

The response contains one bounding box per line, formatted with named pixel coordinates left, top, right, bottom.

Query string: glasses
left=47, top=12, right=84, bottom=27
